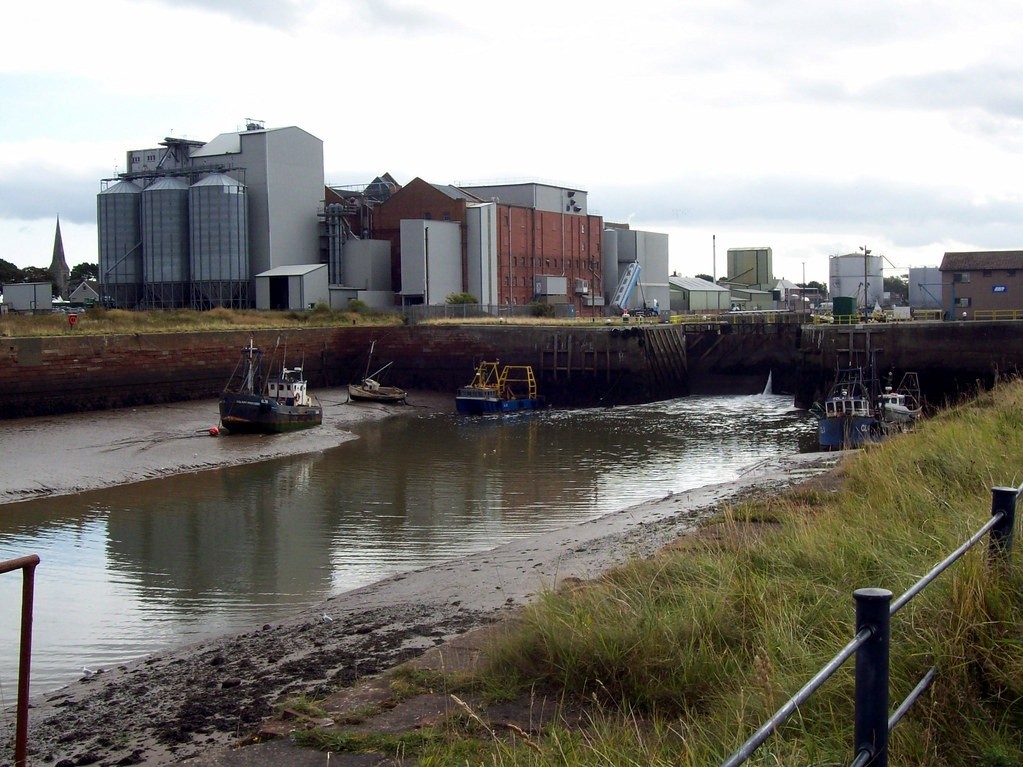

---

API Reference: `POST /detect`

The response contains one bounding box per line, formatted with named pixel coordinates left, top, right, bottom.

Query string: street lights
left=860, top=246, right=872, bottom=323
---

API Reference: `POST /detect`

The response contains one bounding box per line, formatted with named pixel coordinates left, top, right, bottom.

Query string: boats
left=218, top=333, right=324, bottom=431
left=455, top=359, right=547, bottom=414
left=874, top=369, right=925, bottom=423
left=346, top=340, right=408, bottom=403
left=809, top=360, right=886, bottom=452
